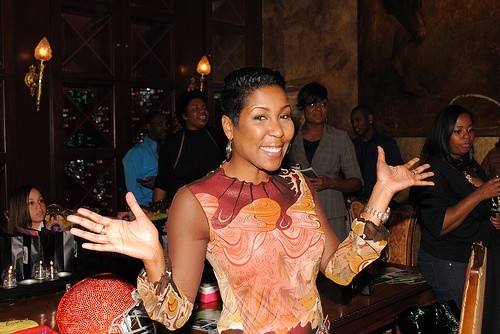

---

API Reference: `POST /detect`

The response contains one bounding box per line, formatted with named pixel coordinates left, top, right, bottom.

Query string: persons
left=152, top=88, right=227, bottom=204
left=120, top=110, right=172, bottom=208
left=346, top=104, right=403, bottom=205
left=413, top=103, right=500, bottom=309
left=285, top=81, right=366, bottom=244
left=0, top=182, right=64, bottom=278
left=66, top=66, right=436, bottom=334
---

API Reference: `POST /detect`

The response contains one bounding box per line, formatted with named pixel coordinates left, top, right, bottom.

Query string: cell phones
left=135, top=177, right=152, bottom=183
left=301, top=168, right=318, bottom=180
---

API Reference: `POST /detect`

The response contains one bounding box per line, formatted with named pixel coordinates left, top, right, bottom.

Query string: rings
left=410, top=170, right=416, bottom=175
left=100, top=226, right=107, bottom=235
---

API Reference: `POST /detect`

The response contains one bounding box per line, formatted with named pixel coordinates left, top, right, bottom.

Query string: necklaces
left=463, top=170, right=475, bottom=185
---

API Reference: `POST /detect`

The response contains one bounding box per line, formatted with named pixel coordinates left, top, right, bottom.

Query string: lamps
left=25, top=37, right=52, bottom=111
left=188, top=55, right=210, bottom=93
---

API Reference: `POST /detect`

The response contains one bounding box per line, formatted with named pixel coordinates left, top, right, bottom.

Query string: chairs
left=458, top=240, right=488, bottom=334
left=55, top=272, right=143, bottom=334
left=348, top=200, right=418, bottom=268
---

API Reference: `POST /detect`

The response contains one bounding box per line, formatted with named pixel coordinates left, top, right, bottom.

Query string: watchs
left=363, top=204, right=391, bottom=224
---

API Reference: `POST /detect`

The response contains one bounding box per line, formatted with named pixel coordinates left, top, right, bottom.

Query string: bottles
left=63, top=88, right=116, bottom=214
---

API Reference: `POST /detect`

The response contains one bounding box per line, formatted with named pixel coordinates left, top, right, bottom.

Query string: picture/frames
left=356, top=1, right=500, bottom=139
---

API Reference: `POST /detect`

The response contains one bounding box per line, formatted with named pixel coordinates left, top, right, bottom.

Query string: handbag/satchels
left=14, top=225, right=40, bottom=279
left=0, top=231, right=25, bottom=281
left=41, top=221, right=75, bottom=273
left=395, top=300, right=460, bottom=334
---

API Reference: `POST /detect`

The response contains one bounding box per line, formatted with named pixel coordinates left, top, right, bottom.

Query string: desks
left=141, top=259, right=449, bottom=334
left=0, top=290, right=67, bottom=334
